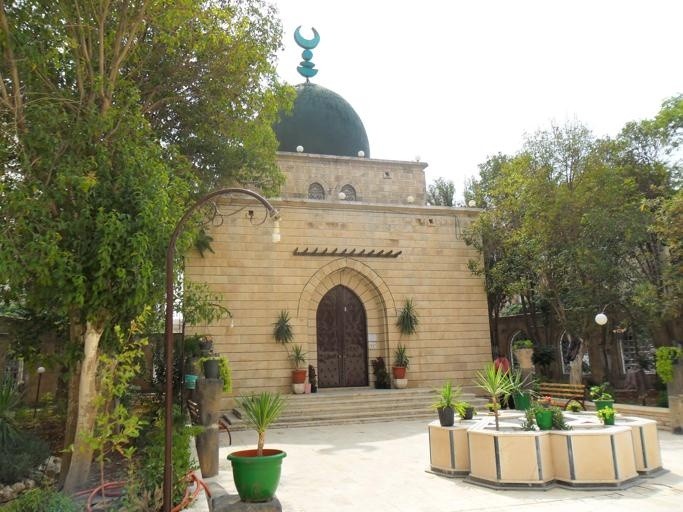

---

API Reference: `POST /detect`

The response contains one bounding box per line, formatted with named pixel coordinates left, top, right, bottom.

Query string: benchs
left=539, top=382, right=587, bottom=411
left=185, top=397, right=231, bottom=445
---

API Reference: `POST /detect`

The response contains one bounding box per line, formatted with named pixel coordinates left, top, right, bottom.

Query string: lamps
left=338, top=192, right=346, bottom=200
left=296, top=145, right=304, bottom=153
left=358, top=151, right=365, bottom=158
left=414, top=154, right=421, bottom=162
left=407, top=196, right=414, bottom=204
left=468, top=199, right=476, bottom=208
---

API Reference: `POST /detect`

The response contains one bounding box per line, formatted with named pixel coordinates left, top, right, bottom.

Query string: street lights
left=31, top=365, right=47, bottom=418
left=649, top=345, right=659, bottom=389
left=162, top=186, right=282, bottom=511
left=594, top=301, right=647, bottom=407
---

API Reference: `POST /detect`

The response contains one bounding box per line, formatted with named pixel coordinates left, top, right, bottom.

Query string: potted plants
left=272, top=308, right=308, bottom=383
left=597, top=405, right=617, bottom=425
left=427, top=380, right=469, bottom=426
left=459, top=402, right=477, bottom=419
left=227, top=390, right=291, bottom=503
left=484, top=403, right=501, bottom=412
left=392, top=295, right=421, bottom=379
left=521, top=401, right=574, bottom=431
left=590, top=381, right=614, bottom=411
left=499, top=365, right=544, bottom=410
left=191, top=355, right=232, bottom=393
left=512, top=339, right=535, bottom=368
left=655, top=345, right=683, bottom=384
left=567, top=400, right=584, bottom=412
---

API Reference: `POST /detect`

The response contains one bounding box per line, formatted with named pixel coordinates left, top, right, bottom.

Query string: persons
left=495, top=352, right=515, bottom=410
